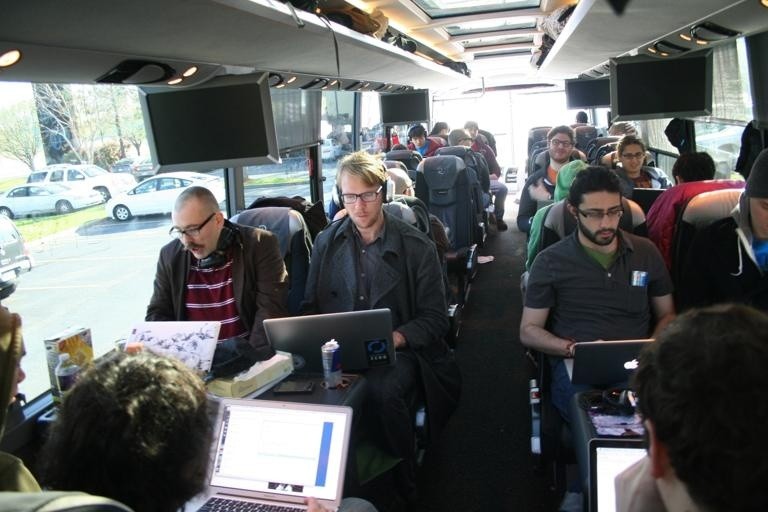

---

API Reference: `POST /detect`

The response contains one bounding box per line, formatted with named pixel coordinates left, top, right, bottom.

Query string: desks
left=39, top=368, right=366, bottom=498
left=570, top=388, right=651, bottom=512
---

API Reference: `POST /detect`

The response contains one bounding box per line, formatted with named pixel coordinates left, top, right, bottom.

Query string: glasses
left=403, top=182, right=416, bottom=193
left=623, top=152, right=642, bottom=158
left=458, top=137, right=473, bottom=141
left=576, top=206, right=625, bottom=219
left=548, top=139, right=574, bottom=146
left=168, top=212, right=215, bottom=238
left=340, top=186, right=383, bottom=204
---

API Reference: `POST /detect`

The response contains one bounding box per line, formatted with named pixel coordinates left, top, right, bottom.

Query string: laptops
left=587, top=438, right=648, bottom=512
left=563, top=342, right=655, bottom=383
left=124, top=320, right=222, bottom=382
left=262, top=309, right=397, bottom=377
left=633, top=188, right=670, bottom=215
left=178, top=398, right=353, bottom=511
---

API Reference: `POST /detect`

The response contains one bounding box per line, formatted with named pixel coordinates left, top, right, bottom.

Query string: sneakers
left=496, top=220, right=508, bottom=231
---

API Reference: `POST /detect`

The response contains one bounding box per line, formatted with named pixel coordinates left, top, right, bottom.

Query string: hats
left=744, top=149, right=768, bottom=198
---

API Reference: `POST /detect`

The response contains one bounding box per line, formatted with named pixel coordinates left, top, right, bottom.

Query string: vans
left=26, top=163, right=136, bottom=204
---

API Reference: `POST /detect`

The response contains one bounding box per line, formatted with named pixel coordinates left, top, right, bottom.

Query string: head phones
left=332, top=159, right=395, bottom=205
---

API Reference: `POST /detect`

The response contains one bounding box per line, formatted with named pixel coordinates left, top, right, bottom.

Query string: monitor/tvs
left=378, top=89, right=430, bottom=126
left=609, top=47, right=713, bottom=123
left=565, top=77, right=610, bottom=110
left=139, top=72, right=282, bottom=173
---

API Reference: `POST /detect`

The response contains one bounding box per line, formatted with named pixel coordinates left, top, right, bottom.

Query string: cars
left=0, top=183, right=104, bottom=218
left=113, top=159, right=132, bottom=174
left=131, top=160, right=160, bottom=175
left=322, top=139, right=343, bottom=162
left=0, top=216, right=32, bottom=300
left=105, top=172, right=225, bottom=221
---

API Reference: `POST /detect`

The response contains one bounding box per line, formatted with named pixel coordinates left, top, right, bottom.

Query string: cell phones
left=274, top=381, right=315, bottom=392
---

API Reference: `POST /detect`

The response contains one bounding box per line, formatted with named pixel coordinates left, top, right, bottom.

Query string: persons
left=405, top=125, right=444, bottom=159
left=524, top=160, right=591, bottom=270
left=465, top=120, right=509, bottom=230
left=516, top=125, right=576, bottom=241
left=299, top=151, right=453, bottom=478
left=518, top=166, right=675, bottom=512
left=608, top=135, right=675, bottom=189
left=574, top=110, right=588, bottom=124
left=614, top=303, right=768, bottom=511
left=609, top=123, right=638, bottom=137
left=0, top=306, right=41, bottom=492
left=1, top=352, right=378, bottom=511
left=428, top=121, right=450, bottom=136
left=147, top=186, right=287, bottom=358
left=672, top=151, right=716, bottom=184
left=682, top=149, right=768, bottom=312
left=434, top=129, right=490, bottom=210
left=382, top=167, right=451, bottom=255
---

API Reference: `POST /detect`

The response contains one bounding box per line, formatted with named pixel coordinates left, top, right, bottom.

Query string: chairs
left=415, top=156, right=481, bottom=306
left=528, top=140, right=547, bottom=171
left=574, top=126, right=596, bottom=151
left=531, top=147, right=546, bottom=170
left=648, top=180, right=751, bottom=266
left=586, top=138, right=622, bottom=166
left=397, top=191, right=463, bottom=344
left=228, top=207, right=312, bottom=363
left=386, top=151, right=422, bottom=183
left=596, top=143, right=656, bottom=171
left=520, top=200, right=646, bottom=504
left=433, top=145, right=494, bottom=244
left=617, top=167, right=672, bottom=191
left=669, top=189, right=767, bottom=266
left=332, top=203, right=462, bottom=500
left=526, top=127, right=553, bottom=148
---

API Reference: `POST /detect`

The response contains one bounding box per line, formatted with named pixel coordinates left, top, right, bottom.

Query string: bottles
left=55, top=354, right=82, bottom=403
left=321, top=340, right=341, bottom=391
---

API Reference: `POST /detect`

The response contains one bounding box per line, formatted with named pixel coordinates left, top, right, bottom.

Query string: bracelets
left=564, top=339, right=573, bottom=358
